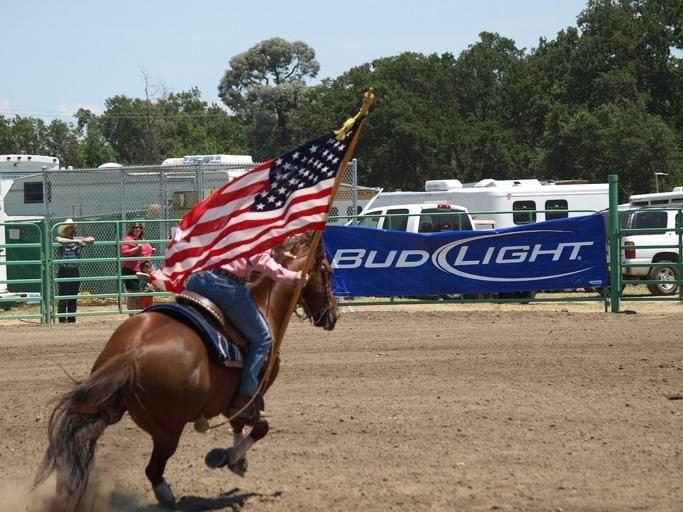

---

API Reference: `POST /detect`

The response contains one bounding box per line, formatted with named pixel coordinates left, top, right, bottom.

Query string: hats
left=58, top=218, right=79, bottom=233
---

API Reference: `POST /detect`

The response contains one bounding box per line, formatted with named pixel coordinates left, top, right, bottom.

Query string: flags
left=146, top=116, right=356, bottom=292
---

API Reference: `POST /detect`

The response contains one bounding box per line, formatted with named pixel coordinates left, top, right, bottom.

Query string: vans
left=343, top=200, right=478, bottom=300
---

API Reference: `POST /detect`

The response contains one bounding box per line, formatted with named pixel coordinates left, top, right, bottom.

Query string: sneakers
left=229, top=394, right=258, bottom=418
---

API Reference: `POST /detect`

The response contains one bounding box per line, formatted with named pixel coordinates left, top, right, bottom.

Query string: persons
left=53, top=218, right=95, bottom=323
left=136, top=260, right=153, bottom=309
left=120, top=221, right=155, bottom=317
left=184, top=172, right=309, bottom=420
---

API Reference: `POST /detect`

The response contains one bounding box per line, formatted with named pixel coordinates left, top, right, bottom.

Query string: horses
left=29, top=228, right=343, bottom=512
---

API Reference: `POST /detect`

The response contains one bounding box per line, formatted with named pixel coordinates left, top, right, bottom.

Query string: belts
left=209, top=265, right=239, bottom=282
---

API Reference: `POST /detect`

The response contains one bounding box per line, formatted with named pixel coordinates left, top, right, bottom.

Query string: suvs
left=587, top=185, right=682, bottom=295
